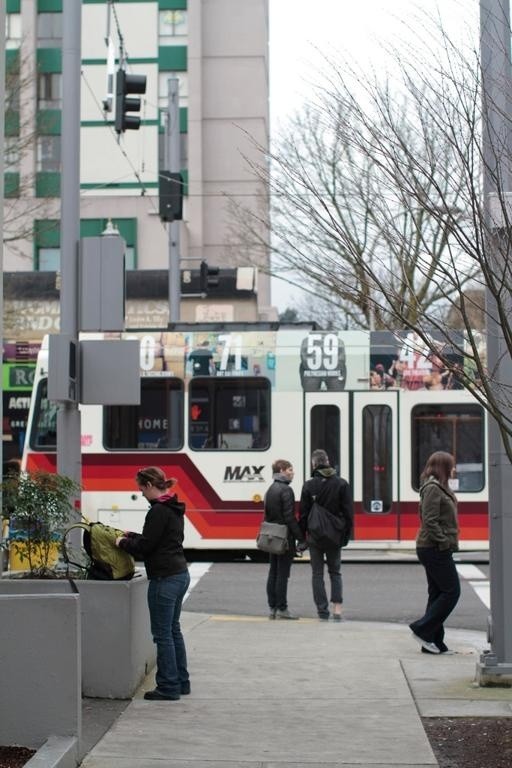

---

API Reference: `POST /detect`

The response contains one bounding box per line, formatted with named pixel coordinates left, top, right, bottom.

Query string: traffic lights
left=199, top=259, right=220, bottom=295
left=114, top=69, right=147, bottom=135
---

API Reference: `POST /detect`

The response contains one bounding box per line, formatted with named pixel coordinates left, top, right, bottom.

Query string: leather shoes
left=269, top=606, right=299, bottom=620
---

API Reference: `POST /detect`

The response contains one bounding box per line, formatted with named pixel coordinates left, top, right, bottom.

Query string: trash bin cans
left=8, top=515, right=61, bottom=573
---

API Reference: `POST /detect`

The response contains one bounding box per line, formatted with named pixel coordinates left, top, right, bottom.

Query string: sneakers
left=143, top=680, right=193, bottom=702
left=317, top=602, right=345, bottom=622
left=408, top=627, right=456, bottom=656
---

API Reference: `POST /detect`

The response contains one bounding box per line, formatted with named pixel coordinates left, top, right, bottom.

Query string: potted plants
left=0, top=471, right=151, bottom=699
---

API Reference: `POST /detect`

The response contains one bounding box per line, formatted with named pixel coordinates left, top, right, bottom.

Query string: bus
left=13, top=326, right=490, bottom=565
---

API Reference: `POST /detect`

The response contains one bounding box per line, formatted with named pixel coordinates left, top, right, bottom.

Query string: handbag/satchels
left=256, top=520, right=290, bottom=556
left=306, top=501, right=348, bottom=548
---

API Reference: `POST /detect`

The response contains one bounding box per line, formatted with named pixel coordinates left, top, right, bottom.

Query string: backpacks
left=59, top=517, right=136, bottom=583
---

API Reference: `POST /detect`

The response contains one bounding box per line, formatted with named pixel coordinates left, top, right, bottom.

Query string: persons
left=2, top=458, right=22, bottom=516
left=295, top=447, right=356, bottom=622
left=189, top=340, right=219, bottom=378
left=262, top=458, right=310, bottom=622
left=408, top=451, right=464, bottom=656
left=115, top=465, right=193, bottom=701
left=369, top=332, right=463, bottom=393
